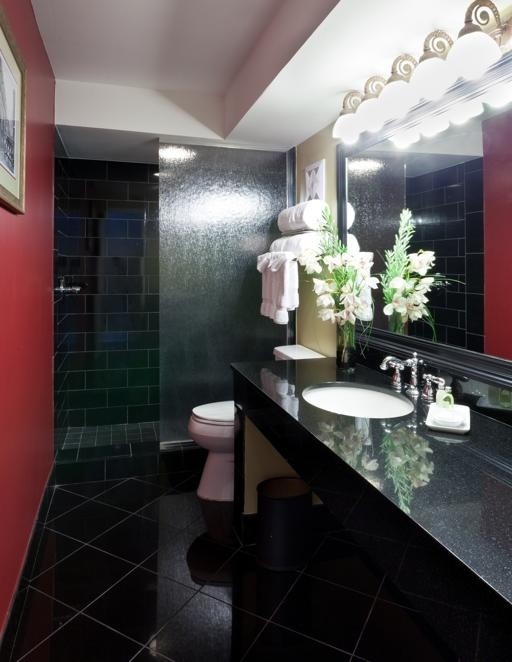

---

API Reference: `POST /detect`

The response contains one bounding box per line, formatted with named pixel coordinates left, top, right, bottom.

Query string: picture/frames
left=305, top=158, right=326, bottom=202
left=0, top=11, right=29, bottom=216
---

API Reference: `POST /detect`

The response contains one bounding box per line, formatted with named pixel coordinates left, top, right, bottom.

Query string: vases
left=336, top=343, right=363, bottom=382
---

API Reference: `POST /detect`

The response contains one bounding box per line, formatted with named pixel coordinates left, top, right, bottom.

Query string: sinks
left=299, top=381, right=415, bottom=419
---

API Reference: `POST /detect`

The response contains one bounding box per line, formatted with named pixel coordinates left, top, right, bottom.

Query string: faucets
left=379, top=352, right=424, bottom=395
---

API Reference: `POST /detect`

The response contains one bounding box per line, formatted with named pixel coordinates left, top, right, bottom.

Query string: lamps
left=407, top=29, right=459, bottom=100
left=355, top=76, right=390, bottom=134
left=376, top=52, right=418, bottom=121
left=446, top=1, right=512, bottom=81
left=332, top=91, right=367, bottom=144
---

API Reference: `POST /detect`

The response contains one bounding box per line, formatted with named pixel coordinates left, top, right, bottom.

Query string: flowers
left=380, top=423, right=435, bottom=515
left=318, top=418, right=379, bottom=477
left=312, top=205, right=378, bottom=343
left=378, top=208, right=465, bottom=342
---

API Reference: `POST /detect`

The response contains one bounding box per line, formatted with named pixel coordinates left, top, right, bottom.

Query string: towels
left=256, top=252, right=298, bottom=324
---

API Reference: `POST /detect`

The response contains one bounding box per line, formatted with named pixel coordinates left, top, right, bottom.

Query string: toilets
left=188, top=344, right=327, bottom=501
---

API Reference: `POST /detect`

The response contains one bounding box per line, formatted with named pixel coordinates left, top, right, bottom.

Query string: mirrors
left=335, top=49, right=512, bottom=388
